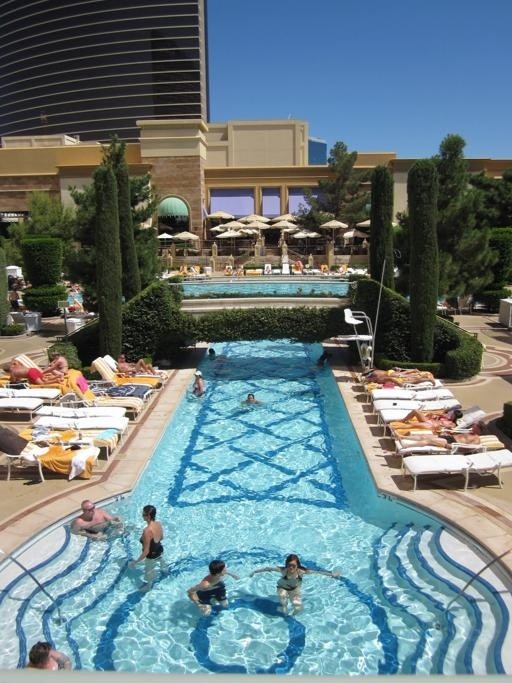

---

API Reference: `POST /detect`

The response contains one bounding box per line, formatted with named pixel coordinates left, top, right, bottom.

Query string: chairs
left=354, top=366, right=512, bottom=492
left=0, top=352, right=169, bottom=483
left=162, top=259, right=367, bottom=280
left=7, top=305, right=97, bottom=335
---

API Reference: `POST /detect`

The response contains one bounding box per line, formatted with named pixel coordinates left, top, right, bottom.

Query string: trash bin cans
left=65, top=318, right=83, bottom=333
left=203, top=267, right=213, bottom=273
left=24, top=312, right=42, bottom=332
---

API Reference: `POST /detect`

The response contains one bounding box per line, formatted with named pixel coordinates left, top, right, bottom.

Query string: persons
left=71, top=500, right=124, bottom=539
left=389, top=408, right=463, bottom=429
left=178, top=256, right=344, bottom=276
left=207, top=347, right=226, bottom=360
left=127, top=505, right=168, bottom=592
left=250, top=553, right=341, bottom=616
left=394, top=420, right=487, bottom=448
left=2, top=361, right=66, bottom=383
left=192, top=369, right=205, bottom=398
left=187, top=559, right=240, bottom=618
left=317, top=350, right=329, bottom=367
left=114, top=353, right=159, bottom=375
left=367, top=367, right=420, bottom=377
left=9, top=276, right=99, bottom=315
left=371, top=373, right=436, bottom=385
left=437, top=293, right=475, bottom=314
left=26, top=640, right=72, bottom=670
left=241, top=394, right=262, bottom=403
left=42, top=351, right=69, bottom=376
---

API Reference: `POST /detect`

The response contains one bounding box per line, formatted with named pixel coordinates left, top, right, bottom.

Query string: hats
left=194, top=371, right=202, bottom=376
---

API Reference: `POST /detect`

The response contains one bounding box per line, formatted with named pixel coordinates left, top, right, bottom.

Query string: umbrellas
left=158, top=210, right=371, bottom=253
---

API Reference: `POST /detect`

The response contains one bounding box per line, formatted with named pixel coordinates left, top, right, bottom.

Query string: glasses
left=287, top=563, right=297, bottom=568
left=142, top=514, right=148, bottom=516
left=84, top=505, right=96, bottom=511
